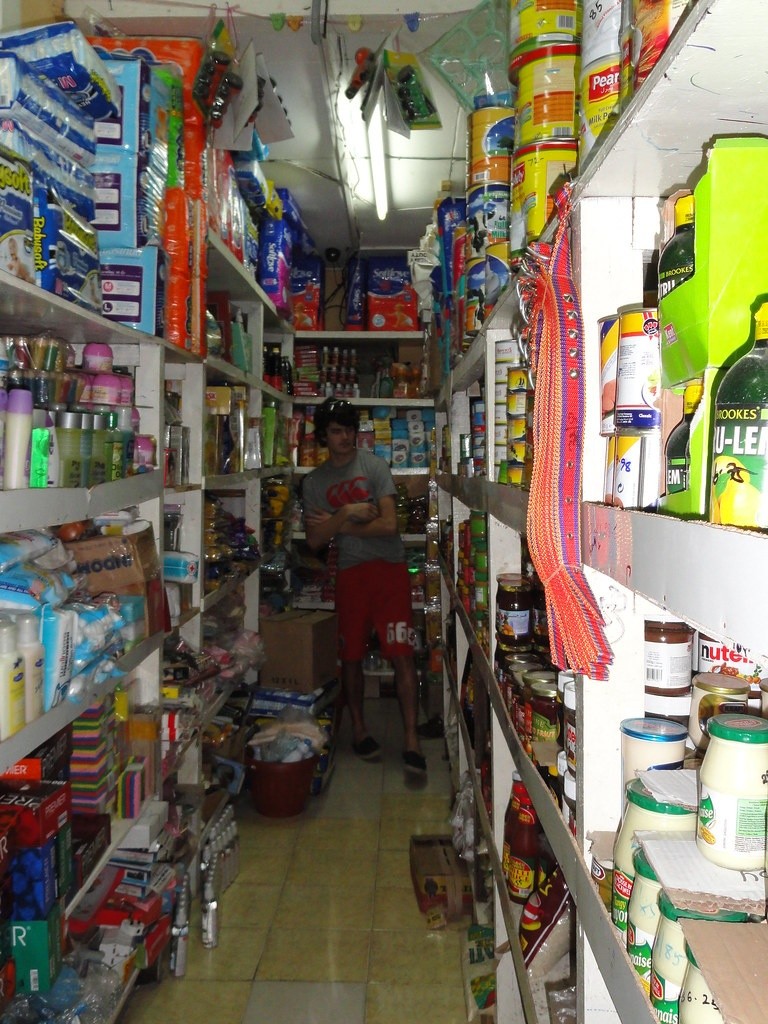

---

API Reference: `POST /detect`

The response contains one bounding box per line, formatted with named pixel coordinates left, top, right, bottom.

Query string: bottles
left=449, top=182, right=768, bottom=1024
left=56, top=406, right=112, bottom=491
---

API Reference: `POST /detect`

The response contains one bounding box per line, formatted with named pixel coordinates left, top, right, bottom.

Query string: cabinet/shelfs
left=1, top=205, right=273, bottom=1024
left=289, top=323, right=437, bottom=679
left=419, top=1, right=766, bottom=1022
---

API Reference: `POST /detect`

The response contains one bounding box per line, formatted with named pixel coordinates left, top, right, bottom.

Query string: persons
left=300, top=395, right=426, bottom=785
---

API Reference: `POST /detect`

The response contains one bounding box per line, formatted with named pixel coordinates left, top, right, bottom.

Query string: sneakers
left=352, top=735, right=382, bottom=759
left=403, top=751, right=426, bottom=774
